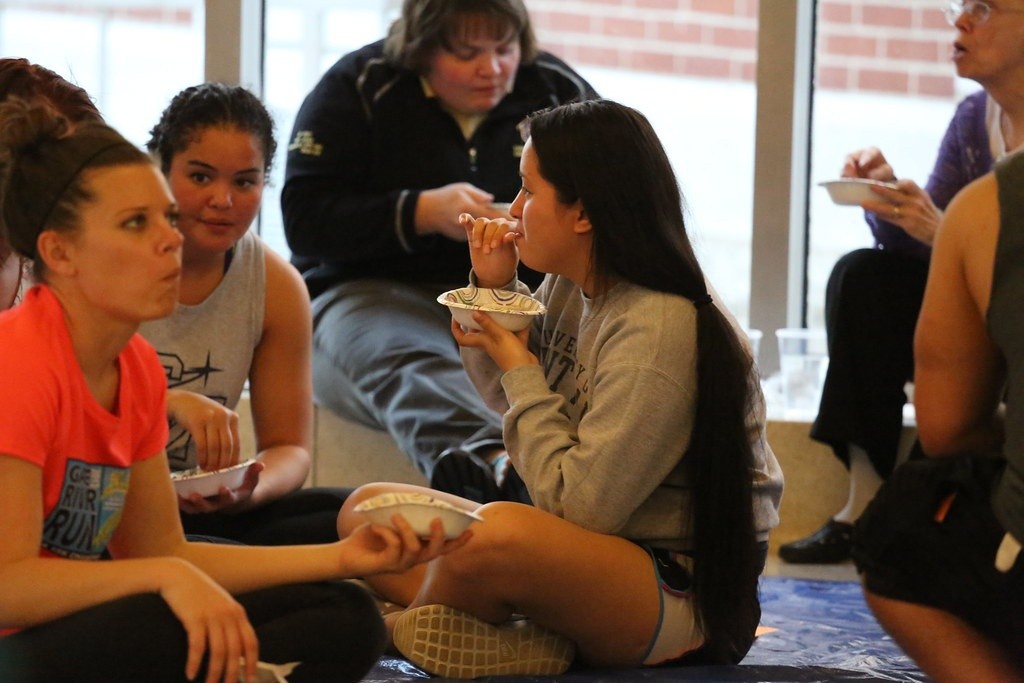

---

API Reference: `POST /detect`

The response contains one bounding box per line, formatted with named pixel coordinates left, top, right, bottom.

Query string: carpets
left=356, top=570, right=931, bottom=683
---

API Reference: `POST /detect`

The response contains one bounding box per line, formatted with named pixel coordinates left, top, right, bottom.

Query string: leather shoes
left=777, top=516, right=860, bottom=565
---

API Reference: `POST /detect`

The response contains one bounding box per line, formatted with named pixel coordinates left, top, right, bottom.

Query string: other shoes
left=393, top=603, right=578, bottom=678
left=494, top=457, right=533, bottom=508
left=427, top=443, right=501, bottom=506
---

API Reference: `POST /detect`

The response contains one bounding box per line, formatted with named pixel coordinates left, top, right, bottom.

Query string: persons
left=0, top=101, right=472, bottom=683
left=851, top=148, right=1024, bottom=683
left=138, top=83, right=361, bottom=547
left=336, top=99, right=785, bottom=680
left=280, top=0, right=605, bottom=506
left=0, top=57, right=104, bottom=314
left=779, top=0, right=1024, bottom=564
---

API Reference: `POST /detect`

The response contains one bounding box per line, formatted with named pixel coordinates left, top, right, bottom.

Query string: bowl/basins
left=354, top=493, right=482, bottom=539
left=437, top=287, right=548, bottom=332
left=171, top=458, right=258, bottom=498
left=817, top=178, right=896, bottom=206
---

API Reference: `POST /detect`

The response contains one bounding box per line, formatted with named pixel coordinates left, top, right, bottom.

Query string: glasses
left=939, top=1, right=1024, bottom=28
